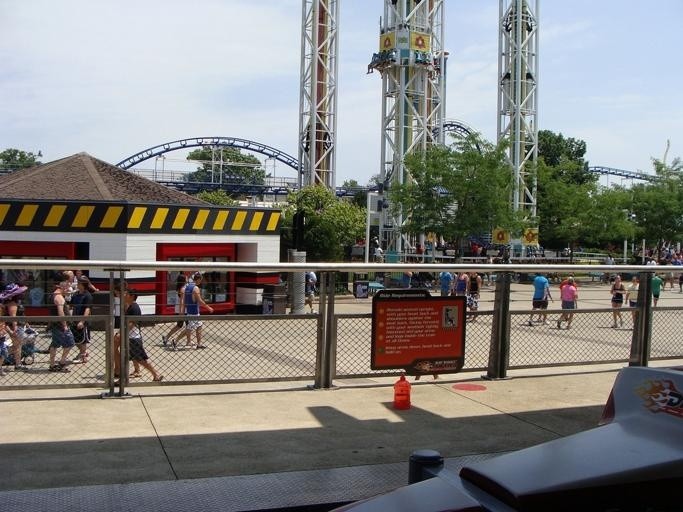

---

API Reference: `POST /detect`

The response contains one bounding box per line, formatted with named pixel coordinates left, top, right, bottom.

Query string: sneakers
left=162, top=336, right=178, bottom=351
left=527, top=318, right=573, bottom=330
left=72, top=351, right=90, bottom=365
left=48, top=364, right=72, bottom=373
left=13, top=365, right=29, bottom=372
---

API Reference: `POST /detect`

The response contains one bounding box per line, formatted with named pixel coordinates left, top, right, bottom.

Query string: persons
left=356, top=234, right=498, bottom=326
left=366, top=48, right=396, bottom=79
left=601, top=243, right=682, bottom=329
left=529, top=271, right=550, bottom=325
left=559, top=271, right=576, bottom=321
left=537, top=286, right=554, bottom=322
left=414, top=50, right=436, bottom=71
left=1, top=269, right=164, bottom=387
left=303, top=268, right=317, bottom=314
left=171, top=273, right=213, bottom=350
left=557, top=276, right=579, bottom=329
left=160, top=274, right=194, bottom=345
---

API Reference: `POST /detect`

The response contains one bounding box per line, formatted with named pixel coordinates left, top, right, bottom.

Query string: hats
left=0, top=282, right=28, bottom=302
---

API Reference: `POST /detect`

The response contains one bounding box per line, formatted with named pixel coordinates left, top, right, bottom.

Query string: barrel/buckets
left=394, top=372, right=411, bottom=410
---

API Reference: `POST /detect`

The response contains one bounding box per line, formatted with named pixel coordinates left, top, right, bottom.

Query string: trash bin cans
left=353, top=281, right=368, bottom=298
left=88, top=291, right=110, bottom=330
left=262, top=284, right=287, bottom=314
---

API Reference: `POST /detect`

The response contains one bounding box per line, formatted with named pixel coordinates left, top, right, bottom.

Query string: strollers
left=0, top=325, right=39, bottom=366
